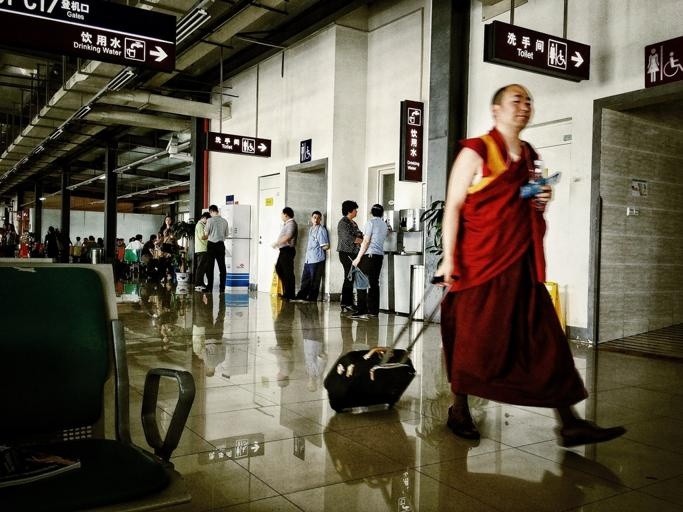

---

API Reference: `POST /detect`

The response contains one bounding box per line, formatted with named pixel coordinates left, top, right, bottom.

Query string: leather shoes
left=446, top=406, right=480, bottom=439
left=559, top=418, right=626, bottom=448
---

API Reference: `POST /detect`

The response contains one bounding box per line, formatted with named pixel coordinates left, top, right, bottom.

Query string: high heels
left=340, top=305, right=356, bottom=312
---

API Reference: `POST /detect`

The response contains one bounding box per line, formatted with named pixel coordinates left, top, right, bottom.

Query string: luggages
left=324, top=276, right=458, bottom=414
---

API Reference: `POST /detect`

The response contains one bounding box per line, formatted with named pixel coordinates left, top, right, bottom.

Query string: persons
left=436, top=84, right=626, bottom=447
left=348, top=203, right=389, bottom=320
left=337, top=200, right=364, bottom=313
left=0, top=206, right=229, bottom=292
left=291, top=210, right=329, bottom=305
left=272, top=207, right=297, bottom=302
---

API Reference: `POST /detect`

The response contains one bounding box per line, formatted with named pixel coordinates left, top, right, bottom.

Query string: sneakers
left=347, top=310, right=377, bottom=320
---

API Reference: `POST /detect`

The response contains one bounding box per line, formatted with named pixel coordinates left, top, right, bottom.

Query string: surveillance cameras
left=165, top=134, right=179, bottom=153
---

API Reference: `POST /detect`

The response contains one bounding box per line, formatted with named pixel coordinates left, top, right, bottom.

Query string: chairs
left=1, top=261, right=196, bottom=512
